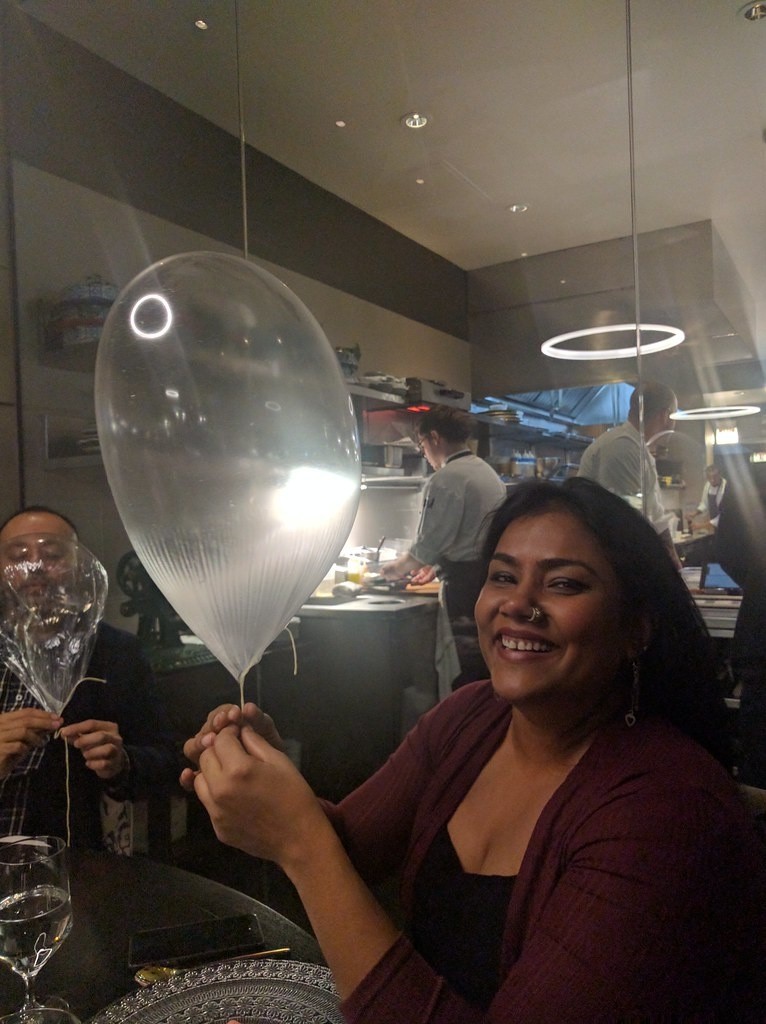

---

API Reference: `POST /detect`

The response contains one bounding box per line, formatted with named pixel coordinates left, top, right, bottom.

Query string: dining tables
left=0, top=838, right=333, bottom=1024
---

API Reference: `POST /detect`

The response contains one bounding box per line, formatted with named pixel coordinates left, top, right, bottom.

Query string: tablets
left=699, top=562, right=744, bottom=596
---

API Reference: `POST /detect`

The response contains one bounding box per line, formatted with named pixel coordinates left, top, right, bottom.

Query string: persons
left=180, top=476, right=766, bottom=1024
left=381, top=406, right=508, bottom=701
left=0, top=506, right=194, bottom=847
left=687, top=465, right=727, bottom=530
left=715, top=470, right=766, bottom=789
left=574, top=381, right=685, bottom=571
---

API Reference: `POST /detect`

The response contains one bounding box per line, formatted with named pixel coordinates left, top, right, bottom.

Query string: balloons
left=93, top=251, right=363, bottom=683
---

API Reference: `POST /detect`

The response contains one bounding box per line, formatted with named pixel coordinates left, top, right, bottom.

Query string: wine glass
left=0, top=835, right=82, bottom=1024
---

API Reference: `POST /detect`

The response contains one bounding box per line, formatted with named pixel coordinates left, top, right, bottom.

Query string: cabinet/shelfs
left=300, top=613, right=439, bottom=751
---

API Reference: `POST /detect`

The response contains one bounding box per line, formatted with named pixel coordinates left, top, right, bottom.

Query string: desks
left=692, top=594, right=743, bottom=638
left=674, top=528, right=714, bottom=566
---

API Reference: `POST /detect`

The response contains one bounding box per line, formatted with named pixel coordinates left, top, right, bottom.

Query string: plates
left=84, top=959, right=347, bottom=1024
left=478, top=410, right=524, bottom=423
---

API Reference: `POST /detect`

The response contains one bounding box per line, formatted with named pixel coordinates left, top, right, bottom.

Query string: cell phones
left=129, top=911, right=264, bottom=968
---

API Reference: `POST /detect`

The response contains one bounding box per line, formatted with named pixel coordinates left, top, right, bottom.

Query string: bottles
left=510, top=448, right=535, bottom=478
left=334, top=558, right=364, bottom=586
